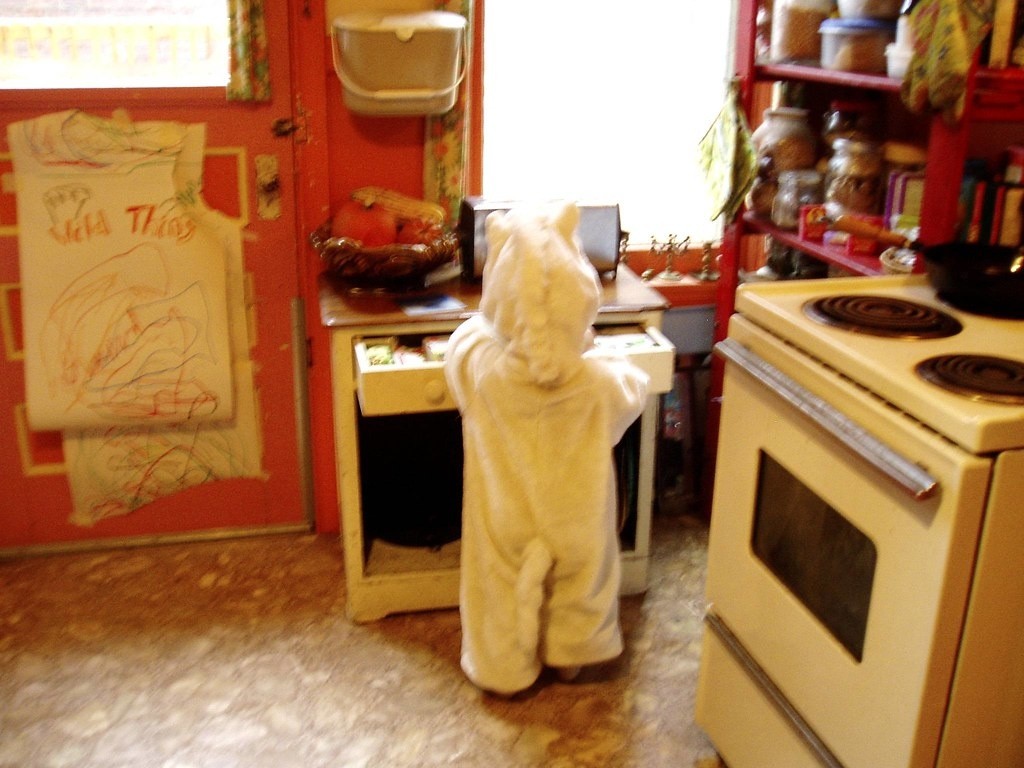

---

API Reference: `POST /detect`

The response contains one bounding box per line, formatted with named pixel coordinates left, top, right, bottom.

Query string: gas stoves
left=733, top=273, right=1024, bottom=453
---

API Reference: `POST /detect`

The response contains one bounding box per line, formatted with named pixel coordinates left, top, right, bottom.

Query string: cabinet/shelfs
left=705, top=0, right=1024, bottom=533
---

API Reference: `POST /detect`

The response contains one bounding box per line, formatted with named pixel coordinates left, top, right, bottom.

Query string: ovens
left=691, top=316, right=992, bottom=768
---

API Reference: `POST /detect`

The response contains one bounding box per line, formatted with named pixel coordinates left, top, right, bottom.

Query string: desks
left=319, top=263, right=677, bottom=623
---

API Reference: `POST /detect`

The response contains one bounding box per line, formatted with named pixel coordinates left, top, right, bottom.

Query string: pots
left=820, top=215, right=1024, bottom=321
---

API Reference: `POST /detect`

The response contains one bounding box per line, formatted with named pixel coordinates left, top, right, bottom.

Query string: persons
left=443, top=205, right=650, bottom=696
